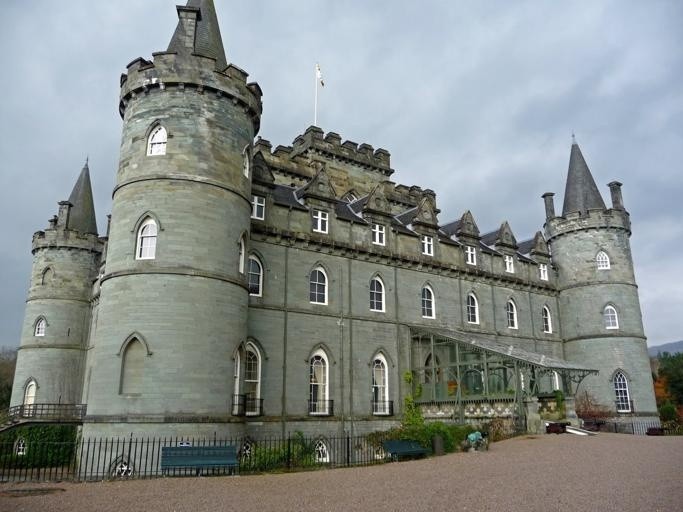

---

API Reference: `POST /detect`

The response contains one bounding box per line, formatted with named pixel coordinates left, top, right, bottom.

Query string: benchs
left=161, top=446, right=240, bottom=478
left=382, top=439, right=433, bottom=462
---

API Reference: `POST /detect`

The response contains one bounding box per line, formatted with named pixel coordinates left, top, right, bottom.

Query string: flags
left=316, top=63, right=324, bottom=87
